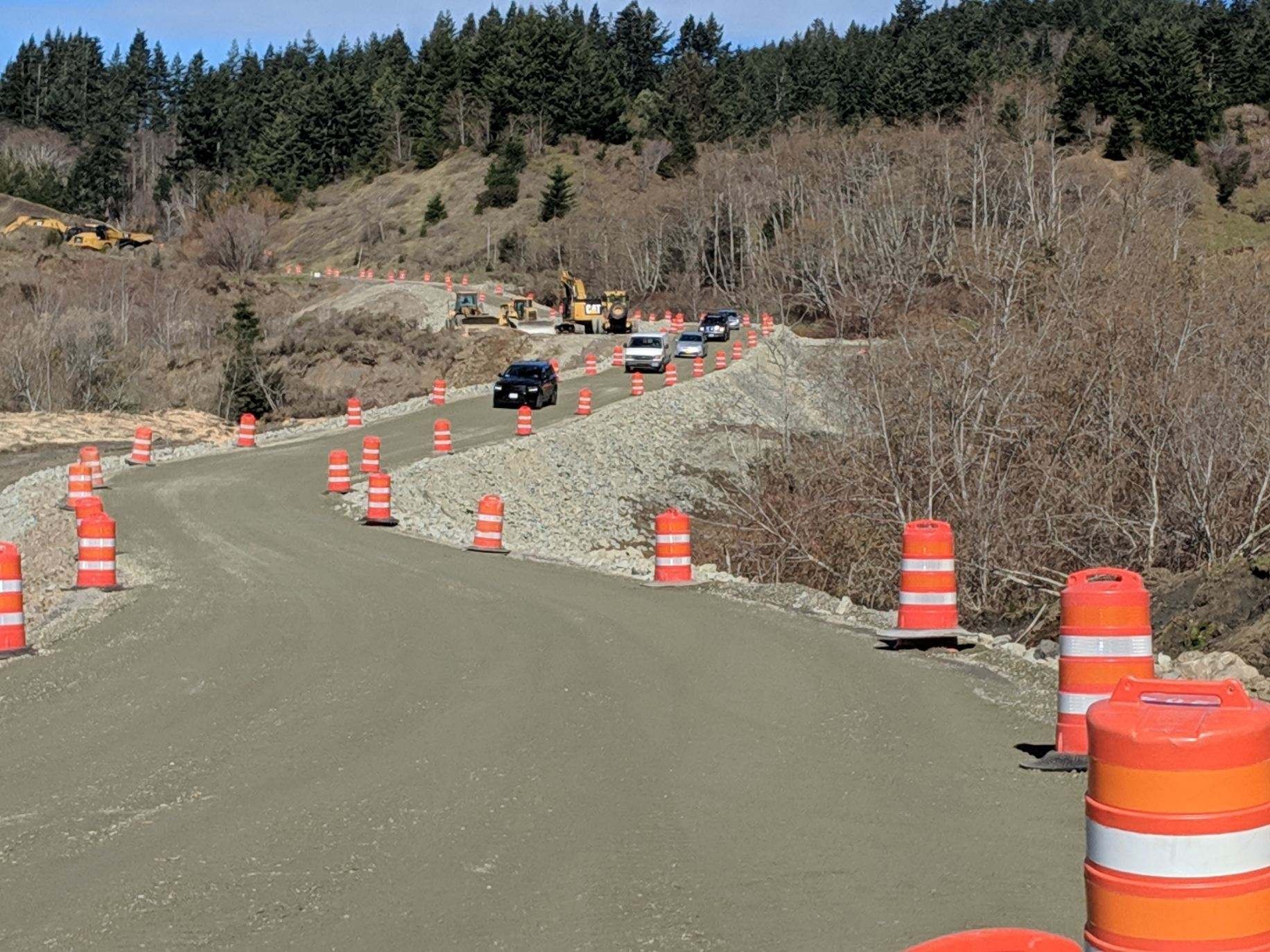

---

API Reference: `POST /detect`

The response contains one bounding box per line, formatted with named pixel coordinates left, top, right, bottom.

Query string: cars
left=622, top=332, right=671, bottom=373
left=493, top=360, right=558, bottom=411
left=674, top=332, right=708, bottom=358
left=718, top=310, right=740, bottom=330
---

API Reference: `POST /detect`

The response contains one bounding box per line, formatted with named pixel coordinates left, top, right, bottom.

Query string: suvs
left=698, top=311, right=730, bottom=342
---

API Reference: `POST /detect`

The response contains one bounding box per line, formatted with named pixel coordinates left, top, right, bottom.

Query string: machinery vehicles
left=554, top=268, right=633, bottom=334
left=0, top=213, right=155, bottom=255
left=499, top=296, right=537, bottom=329
left=445, top=291, right=499, bottom=327
